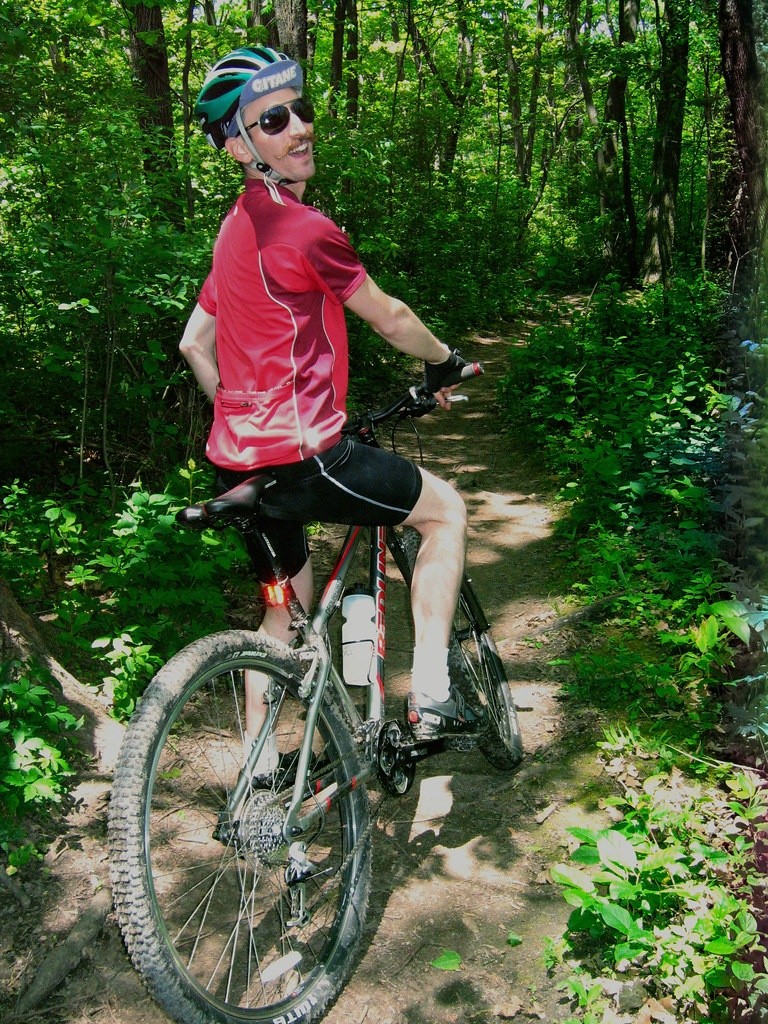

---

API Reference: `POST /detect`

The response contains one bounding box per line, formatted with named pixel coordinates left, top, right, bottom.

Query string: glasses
left=237, top=94, right=316, bottom=144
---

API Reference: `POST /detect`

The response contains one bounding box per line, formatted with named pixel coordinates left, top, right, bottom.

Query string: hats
left=227, top=59, right=304, bottom=141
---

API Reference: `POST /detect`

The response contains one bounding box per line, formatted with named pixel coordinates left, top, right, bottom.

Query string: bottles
left=341, top=582, right=377, bottom=685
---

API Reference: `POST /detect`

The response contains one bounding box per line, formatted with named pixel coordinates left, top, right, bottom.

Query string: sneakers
left=212, top=746, right=318, bottom=847
left=409, top=685, right=488, bottom=741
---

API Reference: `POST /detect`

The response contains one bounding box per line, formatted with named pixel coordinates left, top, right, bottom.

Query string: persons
left=178, top=46, right=490, bottom=792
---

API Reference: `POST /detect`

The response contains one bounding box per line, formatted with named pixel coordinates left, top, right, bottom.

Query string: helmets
left=193, top=47, right=293, bottom=152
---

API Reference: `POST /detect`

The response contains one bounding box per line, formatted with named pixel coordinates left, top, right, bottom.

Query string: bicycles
left=100, top=357, right=526, bottom=1024
